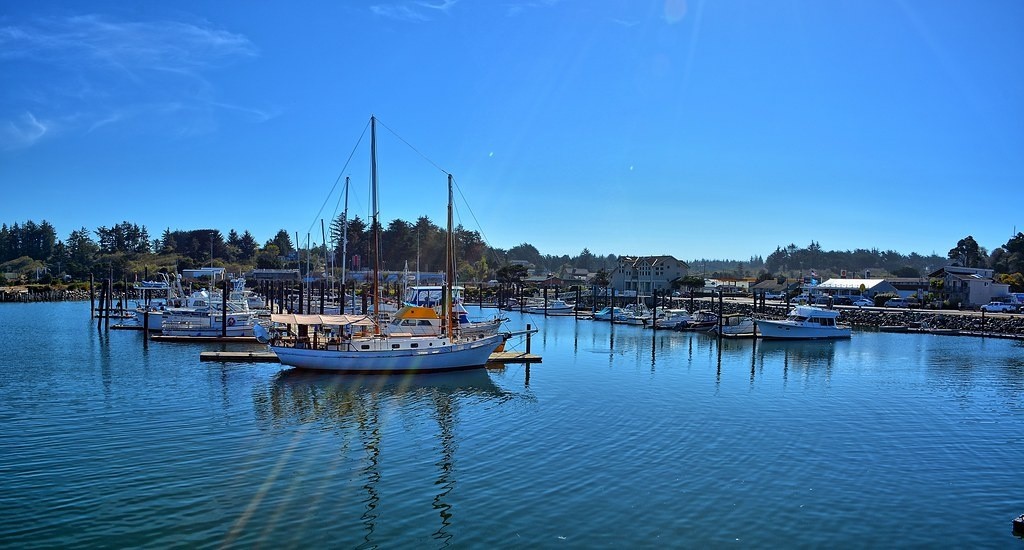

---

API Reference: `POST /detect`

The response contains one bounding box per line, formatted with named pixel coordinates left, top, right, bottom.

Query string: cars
left=853, top=298, right=875, bottom=307
left=670, top=290, right=695, bottom=298
left=790, top=293, right=864, bottom=305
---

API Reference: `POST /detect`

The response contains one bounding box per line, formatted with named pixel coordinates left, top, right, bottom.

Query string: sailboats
left=267, top=115, right=512, bottom=370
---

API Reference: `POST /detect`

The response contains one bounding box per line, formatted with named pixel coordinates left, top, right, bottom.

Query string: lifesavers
left=227, top=317, right=236, bottom=326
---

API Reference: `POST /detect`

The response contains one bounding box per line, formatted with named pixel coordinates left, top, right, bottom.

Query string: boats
left=594, top=303, right=764, bottom=337
left=135, top=291, right=266, bottom=337
left=752, top=304, right=852, bottom=341
left=502, top=297, right=576, bottom=314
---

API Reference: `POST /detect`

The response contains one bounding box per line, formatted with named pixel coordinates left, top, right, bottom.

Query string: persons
left=116, top=302, right=120, bottom=309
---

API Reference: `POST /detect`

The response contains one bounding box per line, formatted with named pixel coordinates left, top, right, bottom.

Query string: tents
left=270, top=314, right=378, bottom=326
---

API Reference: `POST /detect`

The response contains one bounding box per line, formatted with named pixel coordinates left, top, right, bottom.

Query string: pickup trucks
left=755, top=292, right=782, bottom=300
left=979, top=301, right=1016, bottom=313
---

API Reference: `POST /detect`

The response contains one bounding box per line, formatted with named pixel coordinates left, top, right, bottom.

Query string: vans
left=884, top=298, right=908, bottom=308
